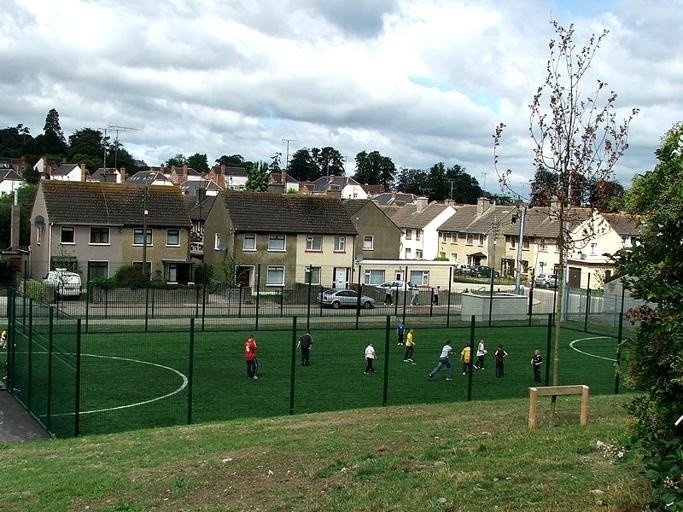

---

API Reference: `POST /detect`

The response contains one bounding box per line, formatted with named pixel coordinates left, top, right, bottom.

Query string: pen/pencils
left=42, top=268, right=82, bottom=297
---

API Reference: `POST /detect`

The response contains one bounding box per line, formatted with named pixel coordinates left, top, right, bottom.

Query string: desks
left=144, top=210, right=148, bottom=277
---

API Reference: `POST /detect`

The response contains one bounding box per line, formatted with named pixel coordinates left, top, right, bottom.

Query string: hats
left=317, top=288, right=376, bottom=308
left=375, top=280, right=413, bottom=291
left=455, top=264, right=499, bottom=278
left=536, top=274, right=561, bottom=288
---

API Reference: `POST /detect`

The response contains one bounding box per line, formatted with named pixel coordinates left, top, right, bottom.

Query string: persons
left=243, top=333, right=258, bottom=381
left=403, top=328, right=416, bottom=363
left=362, top=341, right=377, bottom=376
left=295, top=329, right=313, bottom=366
left=409, top=284, right=419, bottom=306
left=431, top=285, right=441, bottom=305
left=425, top=338, right=453, bottom=381
left=530, top=349, right=543, bottom=385
left=472, top=338, right=488, bottom=371
left=383, top=285, right=393, bottom=305
left=457, top=341, right=471, bottom=375
left=395, top=319, right=405, bottom=346
left=494, top=344, right=508, bottom=377
left=0, top=328, right=8, bottom=350
